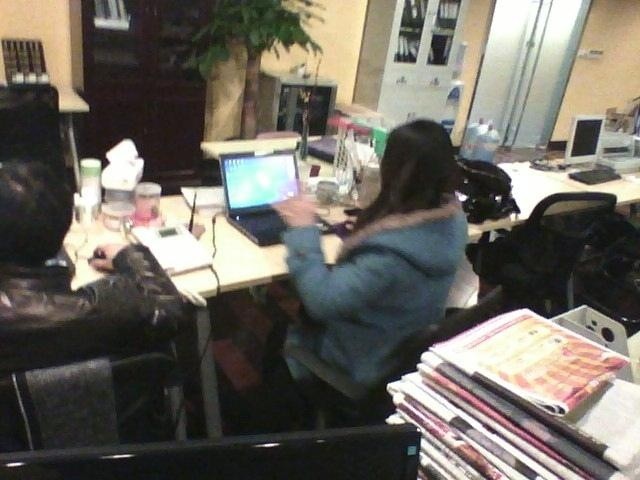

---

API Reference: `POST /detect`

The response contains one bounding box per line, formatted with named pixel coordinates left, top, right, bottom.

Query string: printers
left=598, top=131, right=640, bottom=174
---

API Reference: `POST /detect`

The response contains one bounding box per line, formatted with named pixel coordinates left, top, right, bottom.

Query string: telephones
left=126, top=221, right=214, bottom=308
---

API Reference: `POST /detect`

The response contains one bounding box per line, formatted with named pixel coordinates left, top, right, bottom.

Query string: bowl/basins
left=102, top=202, right=137, bottom=232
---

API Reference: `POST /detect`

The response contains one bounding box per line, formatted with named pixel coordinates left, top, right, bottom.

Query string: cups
left=316, top=181, right=340, bottom=205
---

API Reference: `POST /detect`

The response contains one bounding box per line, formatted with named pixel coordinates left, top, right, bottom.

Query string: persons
left=265, top=119, right=469, bottom=395
left=0, top=159, right=180, bottom=377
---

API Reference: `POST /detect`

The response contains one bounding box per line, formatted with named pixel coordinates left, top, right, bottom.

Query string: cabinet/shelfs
left=350, top=0, right=464, bottom=129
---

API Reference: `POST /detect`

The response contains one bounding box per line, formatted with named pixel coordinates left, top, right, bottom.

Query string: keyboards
left=568, top=168, right=622, bottom=186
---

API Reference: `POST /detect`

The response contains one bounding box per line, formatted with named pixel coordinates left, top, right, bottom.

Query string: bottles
left=458, top=116, right=488, bottom=161
left=471, top=123, right=500, bottom=164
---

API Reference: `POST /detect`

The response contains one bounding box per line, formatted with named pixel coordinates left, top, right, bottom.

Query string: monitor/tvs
left=563, top=114, right=607, bottom=170
left=1, top=422, right=421, bottom=480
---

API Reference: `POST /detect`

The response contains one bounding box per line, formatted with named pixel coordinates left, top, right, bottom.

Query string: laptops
left=218, top=148, right=337, bottom=245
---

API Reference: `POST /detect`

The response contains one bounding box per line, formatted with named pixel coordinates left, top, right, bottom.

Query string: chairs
left=7, top=351, right=166, bottom=452
left=506, top=192, right=619, bottom=311
left=286, top=280, right=506, bottom=415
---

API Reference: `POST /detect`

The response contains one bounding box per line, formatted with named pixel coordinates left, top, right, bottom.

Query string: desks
left=66, top=145, right=640, bottom=438
